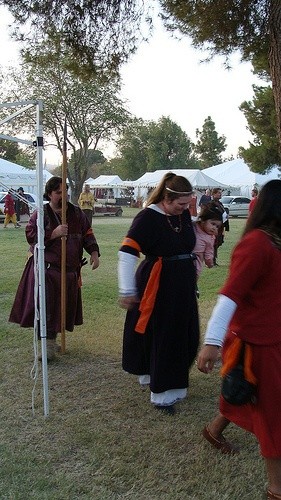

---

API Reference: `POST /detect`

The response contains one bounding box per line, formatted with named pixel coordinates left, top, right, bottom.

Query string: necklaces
left=163, top=204, right=183, bottom=232
left=51, top=203, right=72, bottom=240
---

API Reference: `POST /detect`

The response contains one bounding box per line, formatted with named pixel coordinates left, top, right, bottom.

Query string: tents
left=0, top=156, right=281, bottom=210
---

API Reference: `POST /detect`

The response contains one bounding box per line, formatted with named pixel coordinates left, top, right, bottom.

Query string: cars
left=0, top=193, right=50, bottom=215
left=218, top=196, right=253, bottom=218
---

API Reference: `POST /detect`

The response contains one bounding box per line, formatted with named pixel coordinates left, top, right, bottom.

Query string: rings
left=195, top=360, right=198, bottom=366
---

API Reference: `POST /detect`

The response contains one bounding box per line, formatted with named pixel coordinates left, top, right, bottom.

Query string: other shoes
left=3, top=226, right=8, bottom=228
left=37, top=339, right=60, bottom=361
left=17, top=222, right=21, bottom=226
left=14, top=226, right=20, bottom=228
left=203, top=424, right=237, bottom=454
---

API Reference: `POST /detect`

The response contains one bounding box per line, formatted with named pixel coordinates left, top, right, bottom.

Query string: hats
left=18, top=187, right=25, bottom=192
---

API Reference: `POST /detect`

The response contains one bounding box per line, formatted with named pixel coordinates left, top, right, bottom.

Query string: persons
left=197, top=188, right=259, bottom=265
left=100, top=188, right=105, bottom=199
left=77, top=185, right=95, bottom=228
left=196, top=179, right=281, bottom=500
left=192, top=207, right=222, bottom=298
left=9, top=177, right=100, bottom=362
left=116, top=173, right=200, bottom=417
left=3, top=186, right=29, bottom=229
left=109, top=188, right=114, bottom=199
